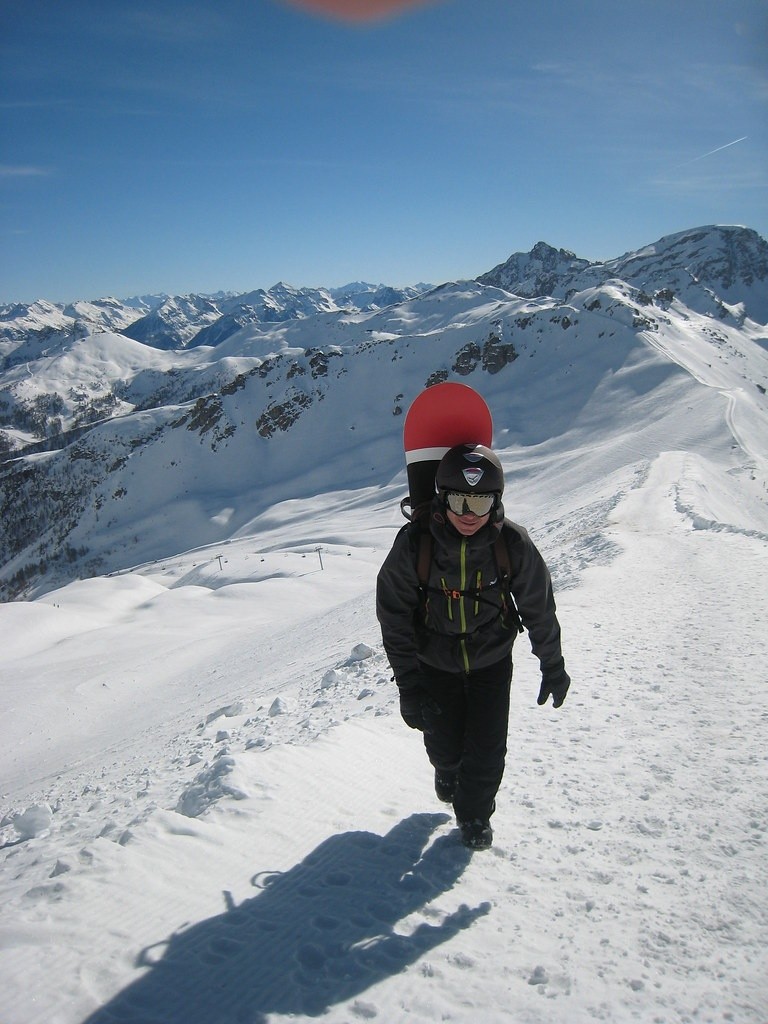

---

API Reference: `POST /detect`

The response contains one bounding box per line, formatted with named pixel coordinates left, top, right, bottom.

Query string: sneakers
left=456, top=817, right=492, bottom=851
left=435, top=768, right=459, bottom=803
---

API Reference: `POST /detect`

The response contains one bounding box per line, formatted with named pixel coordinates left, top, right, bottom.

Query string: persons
left=377, top=443, right=571, bottom=849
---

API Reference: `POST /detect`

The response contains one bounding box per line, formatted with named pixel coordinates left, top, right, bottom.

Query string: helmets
left=434, top=441, right=504, bottom=510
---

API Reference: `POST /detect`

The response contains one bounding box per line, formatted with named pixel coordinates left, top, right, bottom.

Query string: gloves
left=399, top=683, right=442, bottom=736
left=537, top=656, right=570, bottom=708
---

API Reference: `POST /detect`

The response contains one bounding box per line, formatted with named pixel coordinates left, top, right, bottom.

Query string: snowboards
left=402, top=380, right=493, bottom=521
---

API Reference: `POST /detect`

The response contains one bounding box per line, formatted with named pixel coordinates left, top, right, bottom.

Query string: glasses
left=444, top=491, right=496, bottom=518
